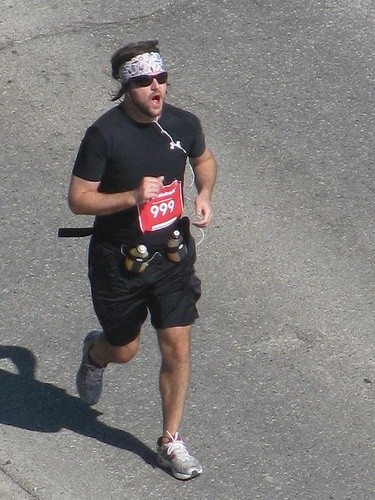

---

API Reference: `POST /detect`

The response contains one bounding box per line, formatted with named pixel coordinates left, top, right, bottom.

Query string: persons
left=67, top=39, right=217, bottom=481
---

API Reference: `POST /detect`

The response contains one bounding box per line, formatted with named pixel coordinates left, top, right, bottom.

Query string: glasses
left=125, top=72, right=169, bottom=89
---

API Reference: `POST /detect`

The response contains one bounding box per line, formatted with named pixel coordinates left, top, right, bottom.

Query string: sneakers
left=76, top=330, right=105, bottom=406
left=156, top=431, right=203, bottom=480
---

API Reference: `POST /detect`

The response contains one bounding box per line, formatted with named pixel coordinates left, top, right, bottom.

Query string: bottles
left=126, top=246, right=149, bottom=272
left=168, top=231, right=188, bottom=262
